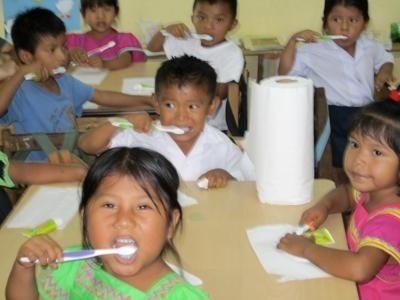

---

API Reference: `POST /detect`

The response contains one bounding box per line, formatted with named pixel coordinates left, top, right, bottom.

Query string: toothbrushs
left=161, top=30, right=214, bottom=42
left=24, top=67, right=67, bottom=81
left=70, top=41, right=116, bottom=67
left=294, top=35, right=348, bottom=40
left=20, top=246, right=138, bottom=263
left=296, top=219, right=316, bottom=235
left=112, top=121, right=185, bottom=135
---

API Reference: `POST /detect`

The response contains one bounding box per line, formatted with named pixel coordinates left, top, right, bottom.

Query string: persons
left=0, top=150, right=87, bottom=189
left=148, top=0, right=245, bottom=135
left=277, top=0, right=396, bottom=169
left=64, top=1, right=148, bottom=70
left=76, top=54, right=255, bottom=190
left=0, top=8, right=154, bottom=134
left=275, top=98, right=400, bottom=300
left=5, top=145, right=210, bottom=300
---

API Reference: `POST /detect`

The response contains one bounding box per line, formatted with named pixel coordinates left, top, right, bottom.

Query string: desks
left=64, top=59, right=168, bottom=116
left=0, top=177, right=359, bottom=300
left=142, top=41, right=400, bottom=84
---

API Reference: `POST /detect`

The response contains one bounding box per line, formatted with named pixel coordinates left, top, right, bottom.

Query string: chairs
left=227, top=62, right=247, bottom=148
left=314, top=87, right=347, bottom=185
left=0, top=129, right=95, bottom=208
